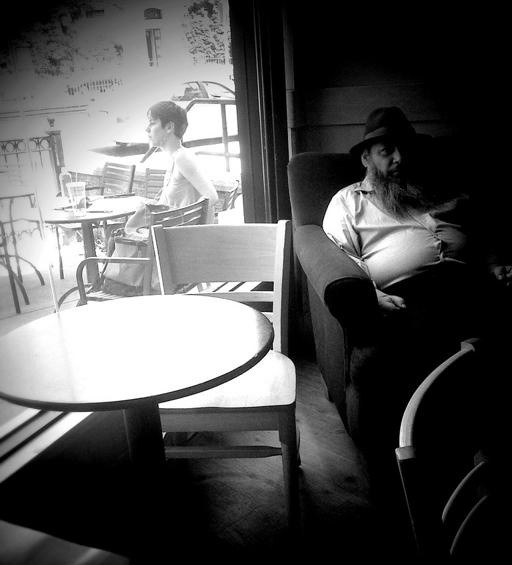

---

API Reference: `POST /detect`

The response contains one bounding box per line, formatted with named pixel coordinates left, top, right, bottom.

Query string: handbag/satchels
left=102, top=202, right=172, bottom=297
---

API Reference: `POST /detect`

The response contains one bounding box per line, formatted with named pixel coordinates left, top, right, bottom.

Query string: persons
left=98, top=100, right=221, bottom=296
left=322, top=104, right=511, bottom=488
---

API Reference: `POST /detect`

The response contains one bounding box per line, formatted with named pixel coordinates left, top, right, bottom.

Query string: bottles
left=58, top=166, right=72, bottom=200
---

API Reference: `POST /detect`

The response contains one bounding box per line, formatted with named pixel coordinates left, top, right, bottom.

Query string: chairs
left=1, top=161, right=211, bottom=314
left=149, top=196, right=212, bottom=296
left=149, top=221, right=305, bottom=494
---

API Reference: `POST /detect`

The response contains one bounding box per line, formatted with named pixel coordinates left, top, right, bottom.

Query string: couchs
left=286, top=152, right=508, bottom=447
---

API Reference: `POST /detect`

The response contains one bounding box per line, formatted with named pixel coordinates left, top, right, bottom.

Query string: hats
left=350, top=107, right=434, bottom=156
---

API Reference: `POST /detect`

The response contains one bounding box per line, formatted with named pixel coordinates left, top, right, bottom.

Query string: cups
left=66, top=180, right=86, bottom=216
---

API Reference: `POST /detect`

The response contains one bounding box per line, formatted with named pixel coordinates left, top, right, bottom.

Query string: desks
left=0, top=296, right=276, bottom=532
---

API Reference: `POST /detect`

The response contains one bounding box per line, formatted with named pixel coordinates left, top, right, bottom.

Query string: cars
left=106, top=76, right=238, bottom=154
